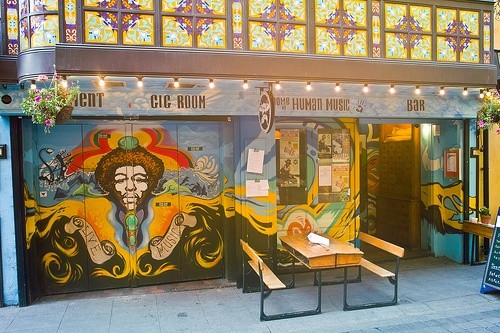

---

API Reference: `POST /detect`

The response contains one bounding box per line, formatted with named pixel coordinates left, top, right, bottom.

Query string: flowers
left=22, top=76, right=81, bottom=132
left=478, top=98, right=500, bottom=136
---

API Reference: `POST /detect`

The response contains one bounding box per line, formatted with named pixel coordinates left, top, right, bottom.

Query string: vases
left=46, top=105, right=73, bottom=125
left=484, top=113, right=500, bottom=123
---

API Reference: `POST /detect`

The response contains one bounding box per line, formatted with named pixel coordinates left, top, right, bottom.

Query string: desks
left=279, top=231, right=365, bottom=315
left=463, top=220, right=495, bottom=265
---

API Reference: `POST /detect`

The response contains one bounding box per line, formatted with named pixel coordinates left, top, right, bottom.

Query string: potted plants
left=481, top=206, right=492, bottom=225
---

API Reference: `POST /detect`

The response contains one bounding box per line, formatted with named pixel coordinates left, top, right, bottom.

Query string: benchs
left=239, top=239, right=320, bottom=321
left=342, top=232, right=405, bottom=311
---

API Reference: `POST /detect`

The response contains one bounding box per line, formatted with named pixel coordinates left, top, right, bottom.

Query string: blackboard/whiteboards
left=482, top=206, right=500, bottom=291
